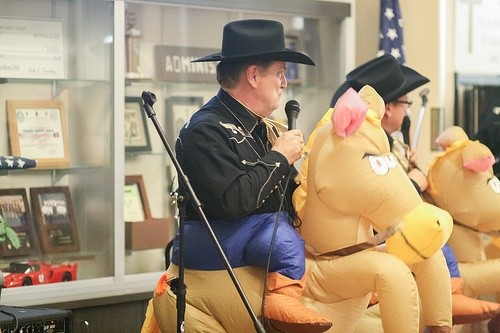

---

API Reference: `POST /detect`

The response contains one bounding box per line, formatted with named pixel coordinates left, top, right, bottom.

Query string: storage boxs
left=124, top=219, right=172, bottom=251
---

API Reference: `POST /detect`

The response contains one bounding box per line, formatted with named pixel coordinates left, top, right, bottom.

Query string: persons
left=174, top=19, right=304, bottom=225
left=330, top=53, right=429, bottom=196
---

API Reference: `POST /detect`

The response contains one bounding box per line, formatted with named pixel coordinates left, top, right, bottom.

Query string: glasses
left=391, top=99, right=413, bottom=108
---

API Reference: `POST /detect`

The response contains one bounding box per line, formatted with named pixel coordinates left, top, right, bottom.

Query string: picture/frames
left=0, top=189, right=37, bottom=257
left=284, top=35, right=302, bottom=85
left=6, top=100, right=70, bottom=167
left=167, top=97, right=202, bottom=147
left=31, top=186, right=82, bottom=253
left=123, top=97, right=151, bottom=151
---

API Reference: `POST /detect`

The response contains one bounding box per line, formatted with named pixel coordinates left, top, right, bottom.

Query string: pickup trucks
left=0, top=261, right=78, bottom=288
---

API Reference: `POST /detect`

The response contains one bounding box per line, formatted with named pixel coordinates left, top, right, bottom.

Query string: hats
left=190, top=18, right=317, bottom=66
left=329, top=54, right=431, bottom=109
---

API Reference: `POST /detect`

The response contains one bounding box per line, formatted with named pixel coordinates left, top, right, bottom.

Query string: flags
left=375, top=0, right=411, bottom=149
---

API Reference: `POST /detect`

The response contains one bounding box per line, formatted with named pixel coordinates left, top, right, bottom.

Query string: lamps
left=126, top=10, right=141, bottom=73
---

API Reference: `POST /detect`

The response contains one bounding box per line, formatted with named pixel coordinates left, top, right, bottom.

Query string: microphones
left=285, top=99, right=301, bottom=131
